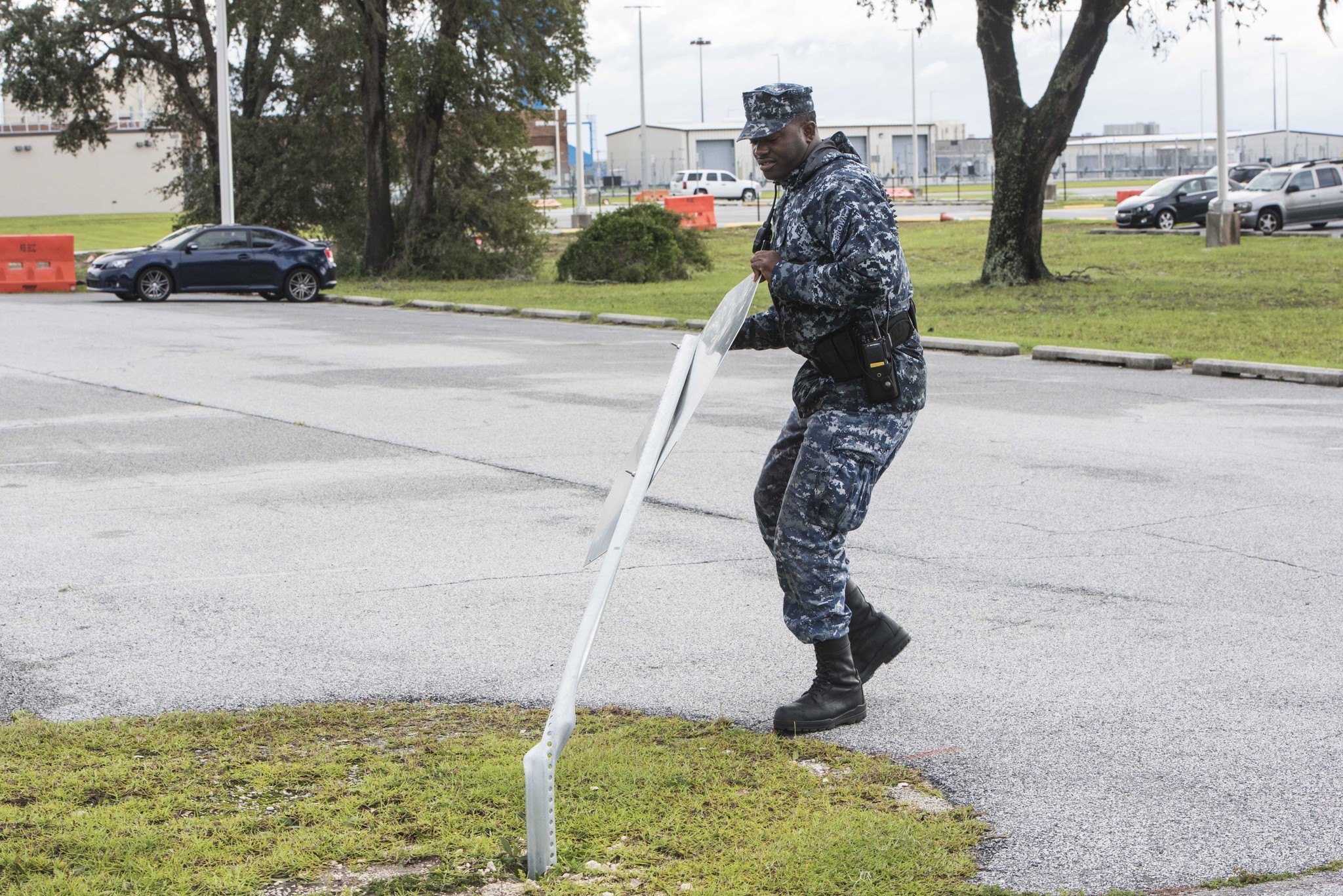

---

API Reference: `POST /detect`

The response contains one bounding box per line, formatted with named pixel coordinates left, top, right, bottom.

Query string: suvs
left=1208, top=157, right=1343, bottom=236
left=670, top=169, right=762, bottom=202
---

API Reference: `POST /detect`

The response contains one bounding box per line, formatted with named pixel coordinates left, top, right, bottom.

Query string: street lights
left=1264, top=34, right=1282, bottom=130
left=690, top=36, right=711, bottom=122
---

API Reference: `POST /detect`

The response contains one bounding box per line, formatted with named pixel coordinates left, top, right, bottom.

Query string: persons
left=1298, top=178, right=1309, bottom=191
left=1184, top=182, right=1193, bottom=194
left=729, top=83, right=929, bottom=733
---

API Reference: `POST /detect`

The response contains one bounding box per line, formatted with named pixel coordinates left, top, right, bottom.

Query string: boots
left=773, top=632, right=869, bottom=734
left=842, top=577, right=912, bottom=687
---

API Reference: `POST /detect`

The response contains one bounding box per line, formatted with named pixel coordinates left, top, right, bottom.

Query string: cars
left=1205, top=161, right=1273, bottom=188
left=86, top=223, right=338, bottom=302
left=1115, top=173, right=1248, bottom=230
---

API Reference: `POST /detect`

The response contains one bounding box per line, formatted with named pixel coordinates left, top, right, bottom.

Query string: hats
left=736, top=82, right=815, bottom=143
